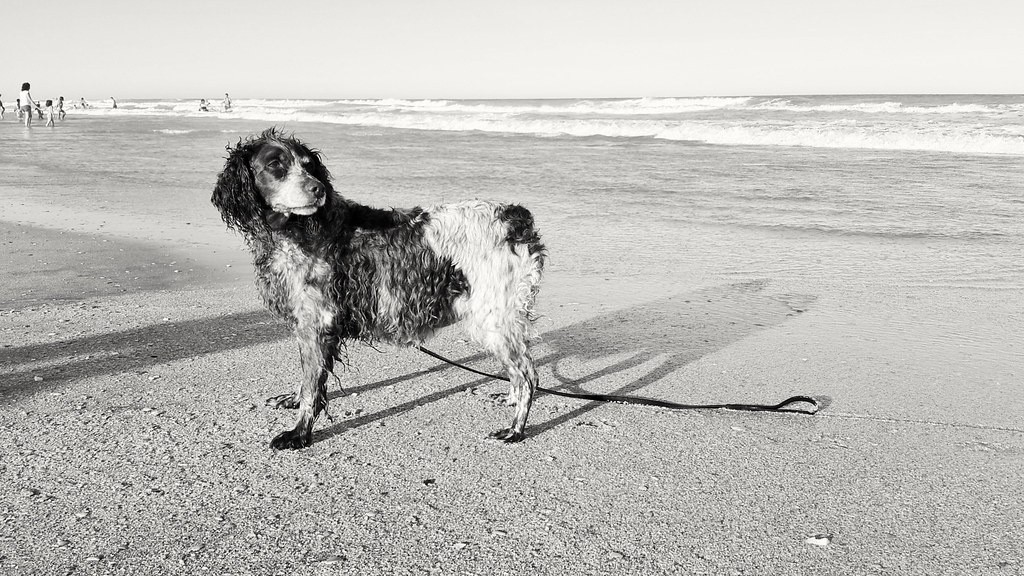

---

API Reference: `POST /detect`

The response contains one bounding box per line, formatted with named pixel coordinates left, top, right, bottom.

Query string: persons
left=57, top=97, right=66, bottom=120
left=199, top=98, right=210, bottom=112
left=111, top=97, right=117, bottom=108
left=38, top=100, right=55, bottom=126
left=0, top=94, right=5, bottom=120
left=222, top=93, right=231, bottom=111
left=74, top=97, right=89, bottom=109
left=20, top=83, right=39, bottom=126
left=35, top=104, right=43, bottom=119
left=14, top=99, right=25, bottom=123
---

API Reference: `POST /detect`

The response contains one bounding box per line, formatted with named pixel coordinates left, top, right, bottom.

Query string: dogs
left=209, top=127, right=545, bottom=452
